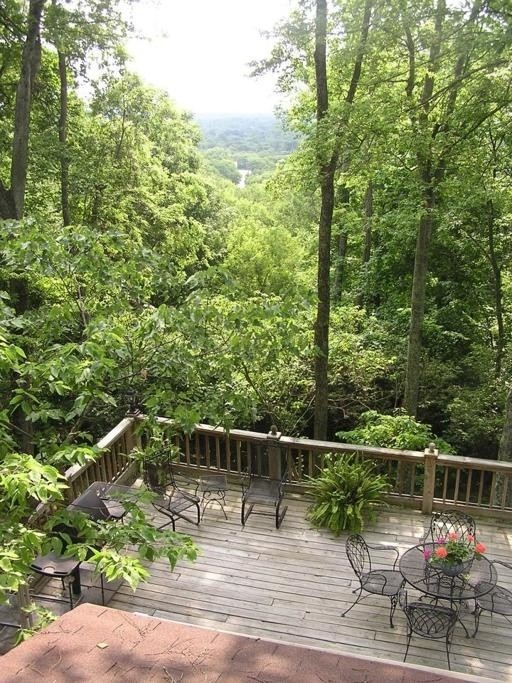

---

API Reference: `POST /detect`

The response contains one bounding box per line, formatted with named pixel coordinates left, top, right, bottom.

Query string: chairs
left=471, top=558, right=511, bottom=638
left=139, top=446, right=201, bottom=533
left=403, top=601, right=459, bottom=670
left=419, top=508, right=476, bottom=597
left=344, top=533, right=406, bottom=627
left=236, top=441, right=290, bottom=529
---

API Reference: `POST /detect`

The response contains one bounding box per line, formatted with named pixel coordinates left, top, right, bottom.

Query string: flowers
left=422, top=531, right=487, bottom=564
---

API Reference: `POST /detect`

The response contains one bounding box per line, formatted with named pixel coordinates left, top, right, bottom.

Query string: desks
left=398, top=541, right=497, bottom=638
left=28, top=481, right=142, bottom=607
left=196, top=474, right=230, bottom=519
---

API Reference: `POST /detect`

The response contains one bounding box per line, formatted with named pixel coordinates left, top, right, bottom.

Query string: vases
left=440, top=558, right=464, bottom=576
